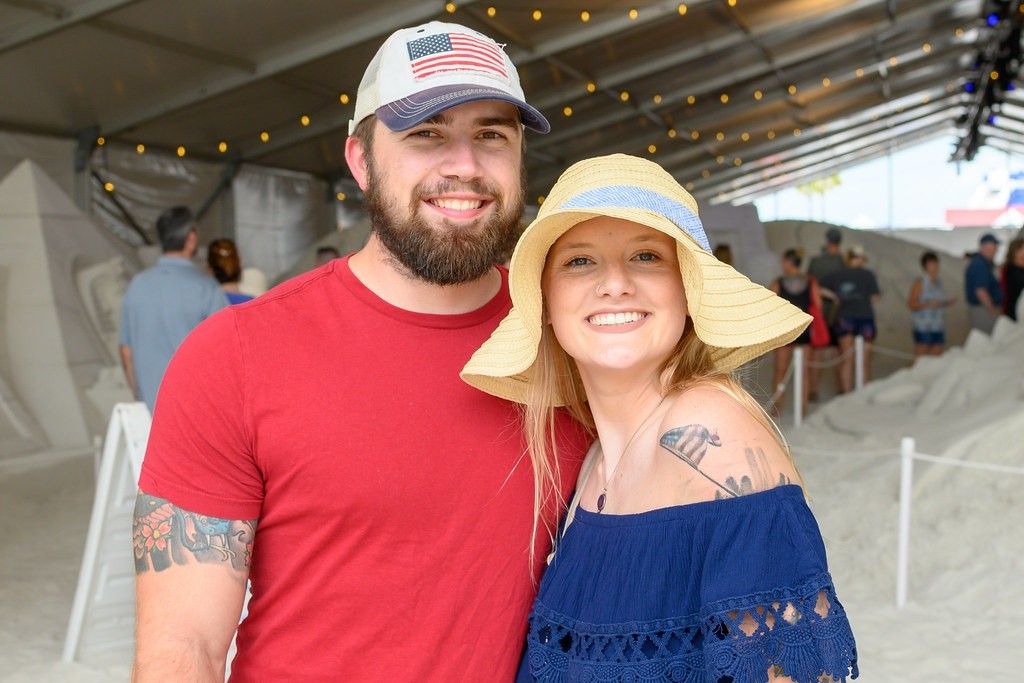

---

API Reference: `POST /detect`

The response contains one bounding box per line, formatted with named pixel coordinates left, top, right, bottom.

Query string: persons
left=118, top=207, right=1023, bottom=420
left=133, top=20, right=600, bottom=680
left=515, top=153, right=859, bottom=682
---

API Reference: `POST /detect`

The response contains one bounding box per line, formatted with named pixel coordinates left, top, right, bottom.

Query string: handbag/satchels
left=808, top=275, right=831, bottom=351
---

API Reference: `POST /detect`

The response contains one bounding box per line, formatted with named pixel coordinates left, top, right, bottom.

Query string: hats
left=347, top=21, right=551, bottom=135
left=848, top=245, right=865, bottom=258
left=459, top=154, right=814, bottom=410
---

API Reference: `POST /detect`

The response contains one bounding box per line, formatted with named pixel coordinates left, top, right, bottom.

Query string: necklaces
left=596, top=389, right=670, bottom=514
